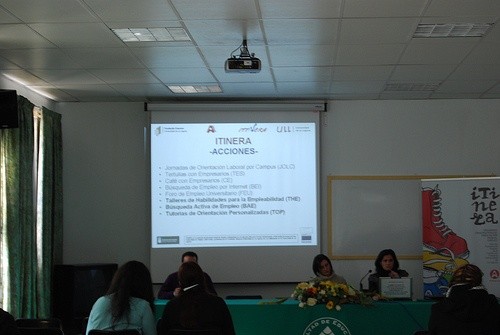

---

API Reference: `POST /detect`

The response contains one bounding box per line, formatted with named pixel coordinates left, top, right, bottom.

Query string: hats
left=448, top=265, right=483, bottom=286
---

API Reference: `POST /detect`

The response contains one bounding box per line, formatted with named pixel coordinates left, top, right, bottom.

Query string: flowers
left=290, top=281, right=387, bottom=311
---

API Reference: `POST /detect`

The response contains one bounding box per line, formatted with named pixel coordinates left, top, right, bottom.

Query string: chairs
left=14, top=318, right=64, bottom=335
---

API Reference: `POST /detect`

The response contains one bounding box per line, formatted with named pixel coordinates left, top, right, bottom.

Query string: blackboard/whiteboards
left=327, top=173, right=423, bottom=257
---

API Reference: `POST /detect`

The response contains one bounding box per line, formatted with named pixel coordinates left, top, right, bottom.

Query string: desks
left=151, top=299, right=440, bottom=335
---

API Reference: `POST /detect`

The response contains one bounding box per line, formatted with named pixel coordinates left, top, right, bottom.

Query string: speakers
left=0, top=90, right=18, bottom=129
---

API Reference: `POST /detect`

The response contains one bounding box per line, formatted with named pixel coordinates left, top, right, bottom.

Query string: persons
left=86, top=261, right=156, bottom=335
left=368, top=249, right=413, bottom=302
left=427, top=264, right=500, bottom=335
left=158, top=251, right=217, bottom=299
left=156, top=261, right=236, bottom=335
left=312, top=254, right=347, bottom=285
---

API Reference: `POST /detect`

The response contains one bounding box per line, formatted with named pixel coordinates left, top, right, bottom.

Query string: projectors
left=225, top=47, right=261, bottom=73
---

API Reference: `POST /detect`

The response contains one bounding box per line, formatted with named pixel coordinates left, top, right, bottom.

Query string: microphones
left=360, top=270, right=372, bottom=289
